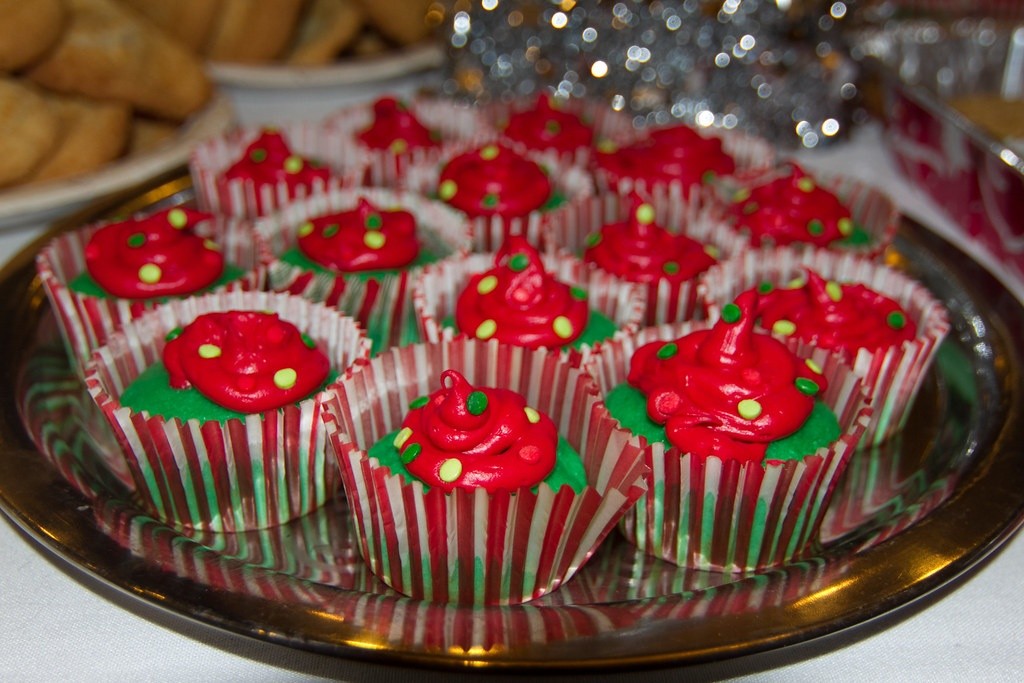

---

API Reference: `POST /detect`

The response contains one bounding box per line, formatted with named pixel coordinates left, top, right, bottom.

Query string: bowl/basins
left=858, top=22, right=1024, bottom=274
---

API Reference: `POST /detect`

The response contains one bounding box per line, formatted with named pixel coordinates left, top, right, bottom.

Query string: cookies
left=0, top=0, right=452, bottom=191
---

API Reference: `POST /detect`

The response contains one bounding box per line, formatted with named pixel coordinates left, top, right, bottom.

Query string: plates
left=201, top=44, right=446, bottom=114
left=0, top=174, right=1022, bottom=665
left=1, top=87, right=237, bottom=228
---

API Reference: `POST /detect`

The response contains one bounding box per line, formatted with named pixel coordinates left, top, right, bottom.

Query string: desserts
left=39, top=82, right=917, bottom=601
